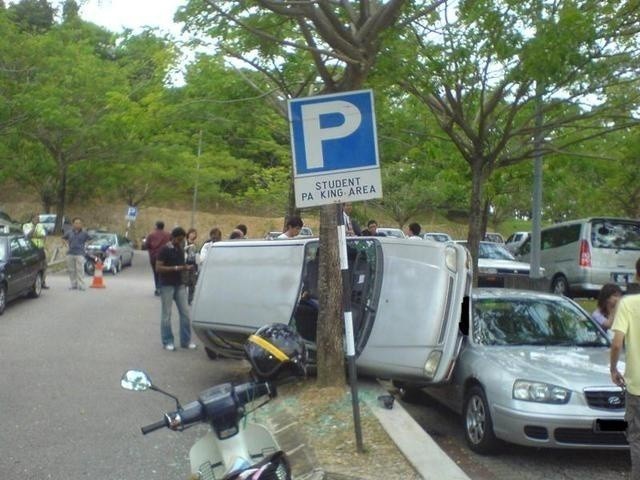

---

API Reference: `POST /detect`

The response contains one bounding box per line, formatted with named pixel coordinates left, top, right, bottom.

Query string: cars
left=1, top=212, right=24, bottom=236
left=84, top=232, right=134, bottom=275
left=23, top=214, right=66, bottom=236
left=0, top=230, right=48, bottom=315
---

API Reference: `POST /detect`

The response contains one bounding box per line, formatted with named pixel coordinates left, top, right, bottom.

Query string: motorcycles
left=120, top=322, right=323, bottom=480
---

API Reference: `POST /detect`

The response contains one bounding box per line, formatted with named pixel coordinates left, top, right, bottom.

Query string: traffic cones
left=91, top=261, right=106, bottom=289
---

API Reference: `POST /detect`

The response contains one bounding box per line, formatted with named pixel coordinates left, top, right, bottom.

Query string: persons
left=145, top=216, right=304, bottom=351
left=23, top=211, right=49, bottom=289
left=589, top=257, right=640, bottom=480
left=340, top=203, right=422, bottom=241
left=61, top=216, right=94, bottom=292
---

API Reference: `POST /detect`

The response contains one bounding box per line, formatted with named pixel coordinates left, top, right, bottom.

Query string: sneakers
left=163, top=342, right=197, bottom=351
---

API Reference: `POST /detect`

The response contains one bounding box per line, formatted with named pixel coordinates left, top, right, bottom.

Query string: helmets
left=242, top=321, right=308, bottom=379
